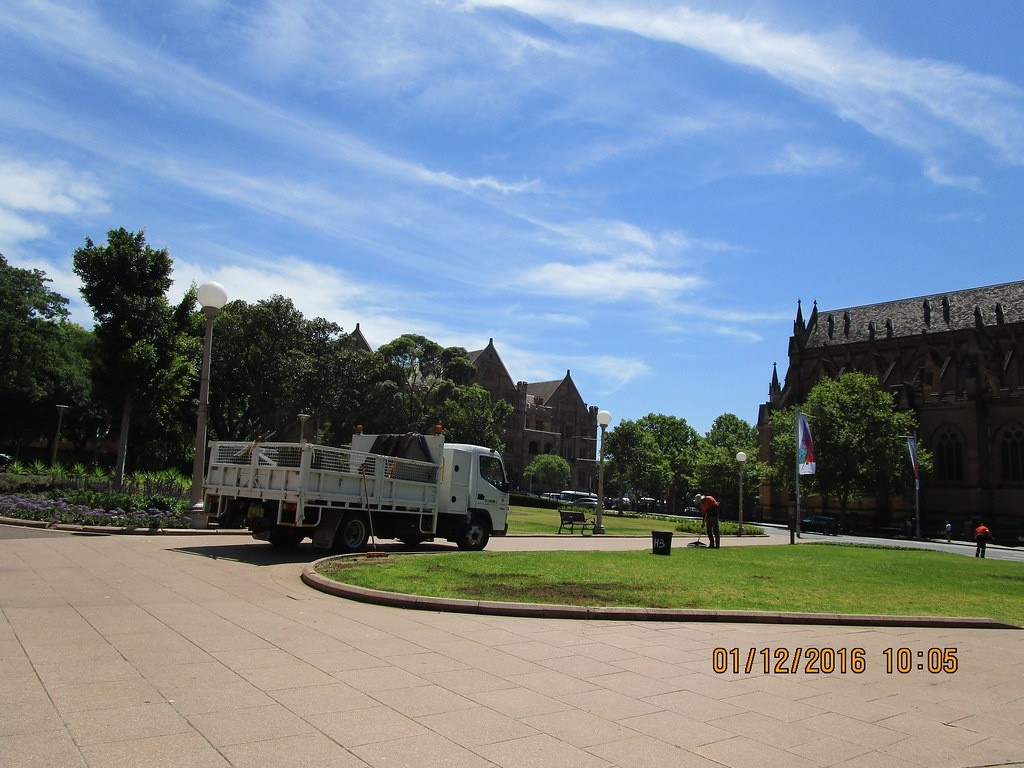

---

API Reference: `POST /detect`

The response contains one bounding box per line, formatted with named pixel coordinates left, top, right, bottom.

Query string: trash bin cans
left=651, top=529, right=674, bottom=555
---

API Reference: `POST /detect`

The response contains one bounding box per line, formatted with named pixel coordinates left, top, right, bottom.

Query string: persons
left=695, top=494, right=720, bottom=549
left=604, top=497, right=611, bottom=509
left=944, top=520, right=952, bottom=540
left=975, top=522, right=995, bottom=558
left=903, top=512, right=916, bottom=539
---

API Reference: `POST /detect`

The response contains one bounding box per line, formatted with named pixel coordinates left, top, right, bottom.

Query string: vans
left=641, top=497, right=656, bottom=508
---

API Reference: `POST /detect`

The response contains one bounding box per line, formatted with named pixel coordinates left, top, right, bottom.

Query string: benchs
left=558, top=509, right=596, bottom=534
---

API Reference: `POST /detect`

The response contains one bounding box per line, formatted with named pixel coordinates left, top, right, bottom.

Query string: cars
left=622, top=498, right=632, bottom=510
left=568, top=498, right=606, bottom=510
left=684, top=507, right=700, bottom=513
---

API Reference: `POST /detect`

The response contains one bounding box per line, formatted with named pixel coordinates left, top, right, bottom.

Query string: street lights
left=735, top=452, right=748, bottom=535
left=297, top=413, right=311, bottom=443
left=594, top=410, right=612, bottom=534
left=184, top=282, right=228, bottom=531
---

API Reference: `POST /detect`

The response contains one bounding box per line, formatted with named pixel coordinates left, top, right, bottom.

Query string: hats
left=694, top=494, right=705, bottom=505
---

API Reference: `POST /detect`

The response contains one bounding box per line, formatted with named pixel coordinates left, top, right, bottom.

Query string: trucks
left=199, top=425, right=515, bottom=553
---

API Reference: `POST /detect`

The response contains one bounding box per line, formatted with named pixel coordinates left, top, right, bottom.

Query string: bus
left=558, top=490, right=597, bottom=504
left=540, top=492, right=561, bottom=501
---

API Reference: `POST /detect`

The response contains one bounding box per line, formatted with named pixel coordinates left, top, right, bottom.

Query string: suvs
left=801, top=516, right=843, bottom=536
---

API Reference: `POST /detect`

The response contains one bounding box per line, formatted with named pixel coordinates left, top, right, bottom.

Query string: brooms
left=687, top=510, right=707, bottom=547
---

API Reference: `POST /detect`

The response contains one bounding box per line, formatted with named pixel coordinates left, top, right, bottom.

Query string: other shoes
left=714, top=547, right=720, bottom=549
left=707, top=546, right=714, bottom=548
